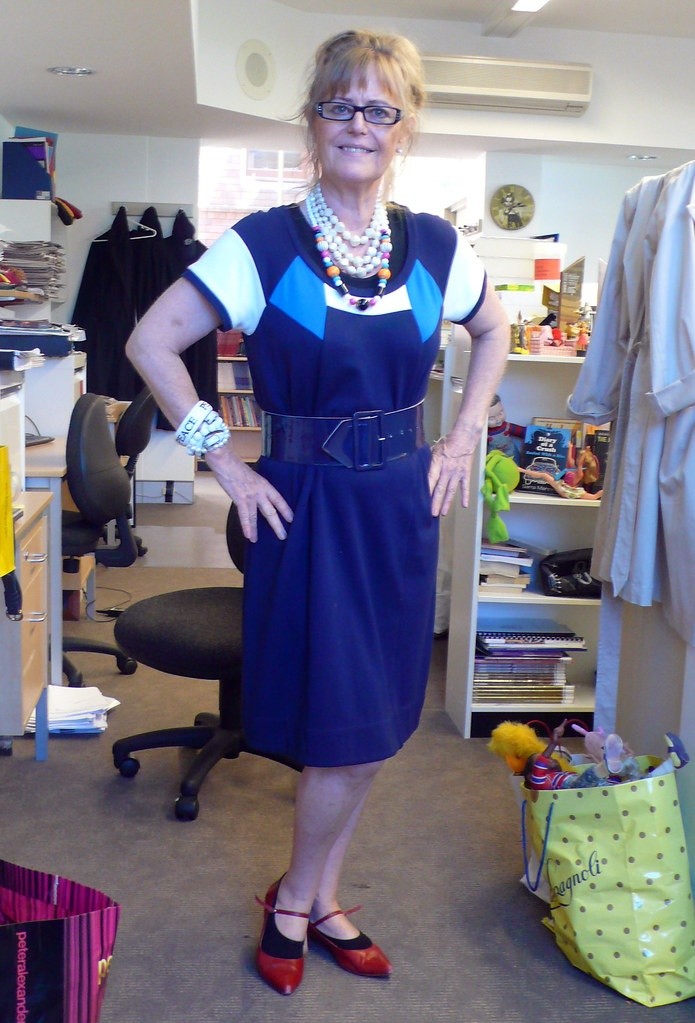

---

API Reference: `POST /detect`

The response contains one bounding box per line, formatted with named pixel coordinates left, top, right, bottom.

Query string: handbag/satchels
left=537, top=546, right=602, bottom=600
left=508, top=718, right=695, bottom=1008
left=0, top=859, right=122, bottom=1023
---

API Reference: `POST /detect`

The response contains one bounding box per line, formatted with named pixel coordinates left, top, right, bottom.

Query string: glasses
left=313, top=101, right=401, bottom=125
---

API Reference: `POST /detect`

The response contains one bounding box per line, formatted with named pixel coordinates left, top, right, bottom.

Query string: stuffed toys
left=573, top=724, right=633, bottom=764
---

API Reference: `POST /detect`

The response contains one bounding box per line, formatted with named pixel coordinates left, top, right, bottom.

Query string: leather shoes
left=306, top=904, right=392, bottom=977
left=255, top=869, right=310, bottom=995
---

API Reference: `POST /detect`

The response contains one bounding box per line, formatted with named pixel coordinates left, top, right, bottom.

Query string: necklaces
left=306, top=184, right=392, bottom=310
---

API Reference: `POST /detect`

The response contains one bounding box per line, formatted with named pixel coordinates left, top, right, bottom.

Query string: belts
left=261, top=397, right=429, bottom=471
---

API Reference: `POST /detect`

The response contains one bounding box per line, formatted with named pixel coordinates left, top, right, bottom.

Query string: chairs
left=59, top=393, right=138, bottom=574
left=101, top=386, right=158, bottom=559
left=112, top=502, right=305, bottom=823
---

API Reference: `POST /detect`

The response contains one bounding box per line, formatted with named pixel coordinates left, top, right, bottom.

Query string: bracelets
left=174, top=401, right=230, bottom=458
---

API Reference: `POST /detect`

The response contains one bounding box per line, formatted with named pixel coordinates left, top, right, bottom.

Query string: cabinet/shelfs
left=198, top=356, right=262, bottom=471
left=0, top=198, right=67, bottom=324
left=442, top=348, right=602, bottom=741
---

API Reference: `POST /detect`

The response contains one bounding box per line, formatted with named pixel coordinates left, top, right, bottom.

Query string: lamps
left=478, top=0, right=550, bottom=39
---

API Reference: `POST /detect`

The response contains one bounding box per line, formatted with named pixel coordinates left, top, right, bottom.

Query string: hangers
left=90, top=205, right=157, bottom=242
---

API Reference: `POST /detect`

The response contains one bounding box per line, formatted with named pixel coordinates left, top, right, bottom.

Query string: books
left=522, top=416, right=612, bottom=497
left=219, top=395, right=262, bottom=427
left=473, top=618, right=588, bottom=704
left=0, top=241, right=86, bottom=371
left=479, top=538, right=556, bottom=593
left=2, top=135, right=52, bottom=200
left=558, top=256, right=599, bottom=329
left=217, top=362, right=253, bottom=390
left=217, top=330, right=246, bottom=357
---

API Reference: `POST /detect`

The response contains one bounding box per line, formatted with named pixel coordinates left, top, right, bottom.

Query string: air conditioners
left=418, top=52, right=593, bottom=119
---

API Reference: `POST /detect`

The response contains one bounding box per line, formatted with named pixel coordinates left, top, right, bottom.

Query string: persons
left=488, top=719, right=689, bottom=789
left=126, top=30, right=510, bottom=994
left=511, top=301, right=592, bottom=350
left=519, top=452, right=603, bottom=499
left=488, top=395, right=527, bottom=462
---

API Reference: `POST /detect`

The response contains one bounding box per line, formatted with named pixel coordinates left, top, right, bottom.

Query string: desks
left=25, top=435, right=68, bottom=685
left=0, top=489, right=56, bottom=762
